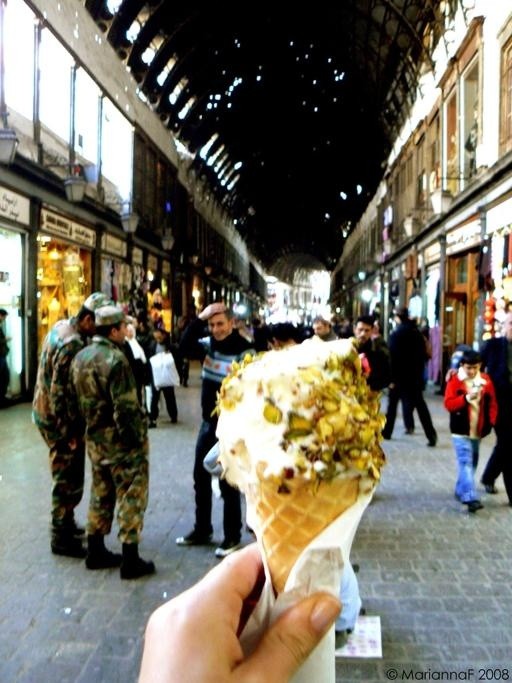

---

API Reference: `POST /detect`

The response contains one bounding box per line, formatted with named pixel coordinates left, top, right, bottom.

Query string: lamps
left=429, top=175, right=470, bottom=215
left=105, top=200, right=140, bottom=232
left=45, top=161, right=87, bottom=202
left=157, top=210, right=177, bottom=251
left=1, top=101, right=20, bottom=167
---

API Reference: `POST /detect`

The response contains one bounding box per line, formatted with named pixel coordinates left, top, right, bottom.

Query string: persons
left=1, top=309, right=10, bottom=403
left=136, top=542, right=342, bottom=682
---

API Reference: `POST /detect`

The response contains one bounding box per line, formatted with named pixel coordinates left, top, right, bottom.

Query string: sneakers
left=467, top=500, right=485, bottom=514
left=215, top=539, right=243, bottom=559
left=175, top=529, right=215, bottom=546
left=51, top=522, right=88, bottom=560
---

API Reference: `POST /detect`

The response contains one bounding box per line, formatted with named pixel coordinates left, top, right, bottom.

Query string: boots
left=85, top=533, right=125, bottom=571
left=120, top=541, right=156, bottom=582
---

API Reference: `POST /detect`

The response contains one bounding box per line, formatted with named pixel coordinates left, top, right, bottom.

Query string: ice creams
left=213, top=338, right=386, bottom=595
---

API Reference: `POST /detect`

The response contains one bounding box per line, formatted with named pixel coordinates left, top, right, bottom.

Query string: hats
left=94, top=305, right=134, bottom=327
left=83, top=291, right=116, bottom=312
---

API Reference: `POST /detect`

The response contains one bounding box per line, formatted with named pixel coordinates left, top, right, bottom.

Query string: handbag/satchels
left=149, top=349, right=182, bottom=392
